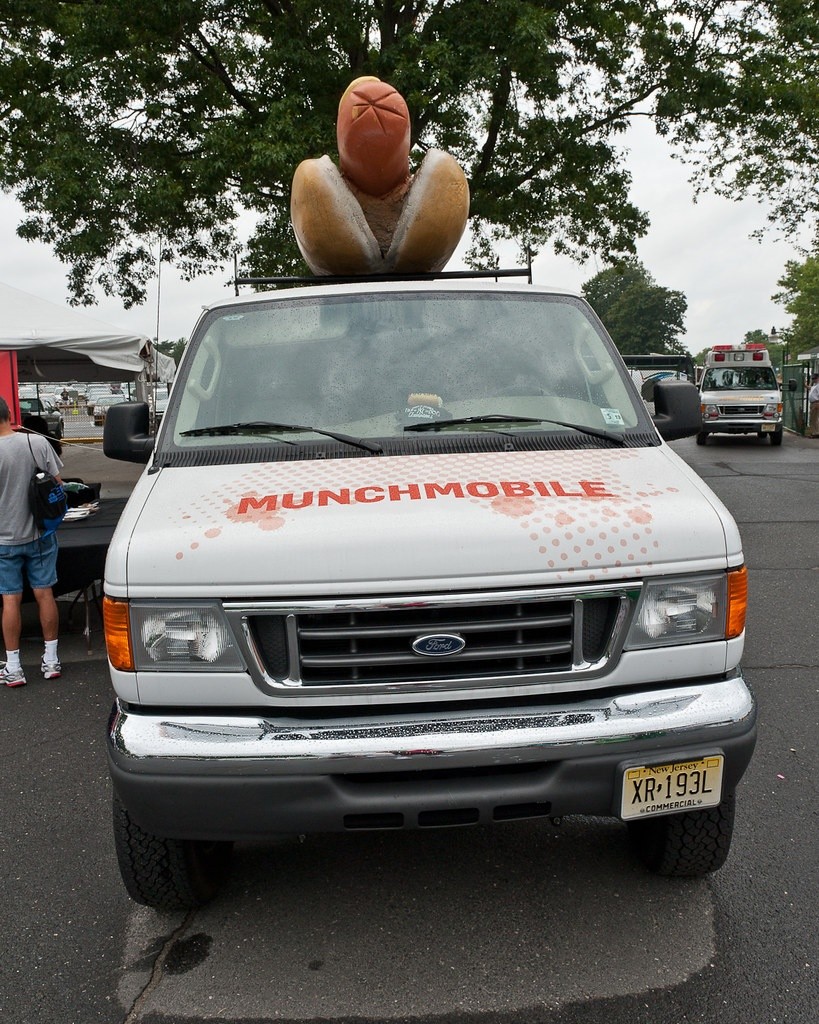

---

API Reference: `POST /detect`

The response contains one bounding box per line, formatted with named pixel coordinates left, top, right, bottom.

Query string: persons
left=60, top=387, right=69, bottom=416
left=804, top=373, right=819, bottom=439
left=0, top=396, right=68, bottom=687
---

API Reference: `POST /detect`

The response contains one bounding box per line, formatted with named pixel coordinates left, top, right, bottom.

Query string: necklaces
left=0, top=428, right=10, bottom=435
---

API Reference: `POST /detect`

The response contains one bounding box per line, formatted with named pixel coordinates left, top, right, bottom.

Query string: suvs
left=101, top=262, right=759, bottom=915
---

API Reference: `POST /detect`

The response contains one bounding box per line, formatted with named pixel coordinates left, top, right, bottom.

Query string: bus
left=579, top=352, right=692, bottom=418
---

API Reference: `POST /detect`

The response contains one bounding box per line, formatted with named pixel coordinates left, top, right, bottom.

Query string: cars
left=18, top=381, right=169, bottom=427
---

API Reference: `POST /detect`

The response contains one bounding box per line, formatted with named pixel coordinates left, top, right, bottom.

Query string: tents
left=0, top=281, right=177, bottom=416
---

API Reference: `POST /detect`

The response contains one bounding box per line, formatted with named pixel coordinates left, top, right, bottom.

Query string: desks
left=54, top=496, right=131, bottom=655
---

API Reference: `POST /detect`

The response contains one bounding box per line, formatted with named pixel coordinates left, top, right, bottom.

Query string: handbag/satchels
left=26, top=432, right=67, bottom=536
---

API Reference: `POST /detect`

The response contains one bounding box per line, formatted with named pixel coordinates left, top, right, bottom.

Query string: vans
left=694, top=344, right=798, bottom=446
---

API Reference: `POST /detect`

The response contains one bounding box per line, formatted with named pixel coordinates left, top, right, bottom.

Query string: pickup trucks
left=19, top=398, right=65, bottom=438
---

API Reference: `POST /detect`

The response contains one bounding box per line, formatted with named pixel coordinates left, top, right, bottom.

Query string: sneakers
left=41, top=654, right=61, bottom=680
left=0, top=661, right=27, bottom=687
